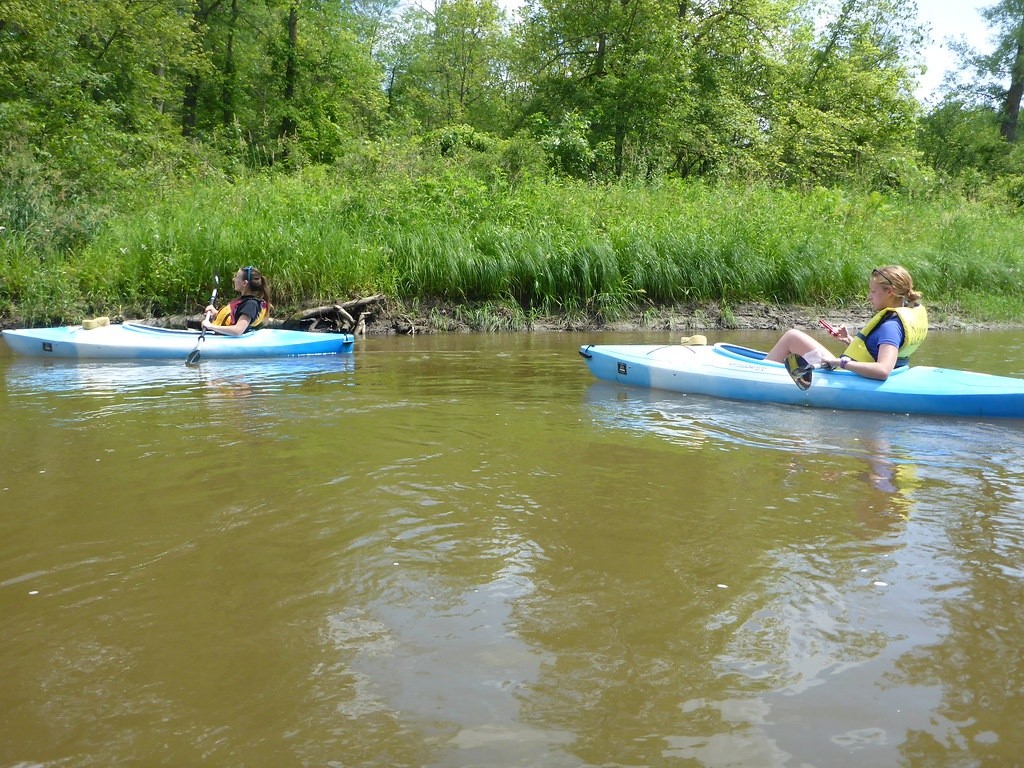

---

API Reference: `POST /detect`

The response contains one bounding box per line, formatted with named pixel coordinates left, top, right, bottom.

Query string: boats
left=2, top=318, right=356, bottom=361
left=578, top=342, right=1024, bottom=420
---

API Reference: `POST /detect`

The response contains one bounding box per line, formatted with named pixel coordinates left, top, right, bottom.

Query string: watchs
left=840, top=356, right=852, bottom=370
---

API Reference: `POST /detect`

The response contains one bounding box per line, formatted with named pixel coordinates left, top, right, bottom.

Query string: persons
left=763, top=264, right=929, bottom=381
left=200, top=266, right=271, bottom=337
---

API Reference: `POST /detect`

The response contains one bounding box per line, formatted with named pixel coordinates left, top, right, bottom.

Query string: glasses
left=871, top=269, right=893, bottom=286
left=244, top=266, right=252, bottom=283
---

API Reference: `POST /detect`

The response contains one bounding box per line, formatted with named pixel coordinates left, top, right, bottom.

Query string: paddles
left=782, top=351, right=828, bottom=392
left=184, top=270, right=221, bottom=366
